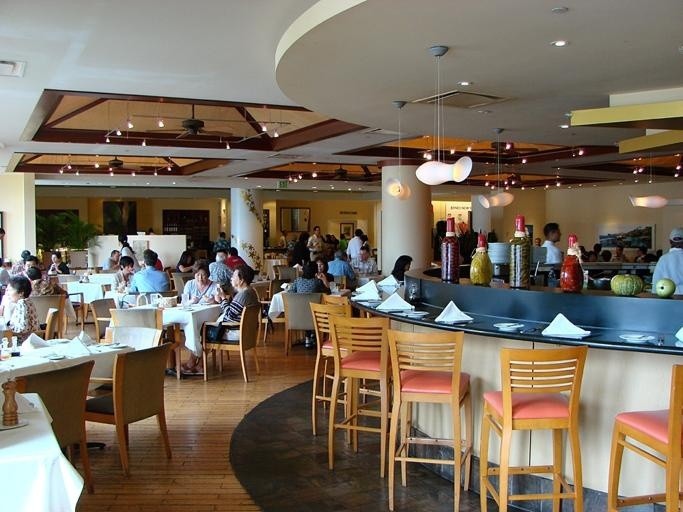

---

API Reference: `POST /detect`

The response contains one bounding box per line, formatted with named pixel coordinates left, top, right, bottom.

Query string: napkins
left=436, top=298, right=474, bottom=323
left=676, top=324, right=682, bottom=345
left=351, top=273, right=415, bottom=312
left=541, top=312, right=591, bottom=338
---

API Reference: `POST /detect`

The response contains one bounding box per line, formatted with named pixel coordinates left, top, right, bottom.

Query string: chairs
left=558, top=248, right=675, bottom=265
left=171, top=244, right=377, bottom=382
left=0, top=234, right=173, bottom=512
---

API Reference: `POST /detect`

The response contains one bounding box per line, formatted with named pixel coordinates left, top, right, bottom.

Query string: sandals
left=175, top=363, right=204, bottom=376
left=305, top=334, right=317, bottom=349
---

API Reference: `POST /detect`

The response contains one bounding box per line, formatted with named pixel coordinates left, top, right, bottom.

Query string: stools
left=308, top=300, right=368, bottom=438
left=479, top=345, right=587, bottom=512
left=606, top=364, right=683, bottom=511
left=325, top=317, right=393, bottom=479
left=387, top=327, right=473, bottom=512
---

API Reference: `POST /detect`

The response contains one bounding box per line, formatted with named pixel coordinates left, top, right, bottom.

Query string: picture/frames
left=594, top=220, right=657, bottom=251
left=338, top=222, right=355, bottom=240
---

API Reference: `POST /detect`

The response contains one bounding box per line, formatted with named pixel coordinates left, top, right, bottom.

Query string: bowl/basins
left=161, top=296, right=178, bottom=308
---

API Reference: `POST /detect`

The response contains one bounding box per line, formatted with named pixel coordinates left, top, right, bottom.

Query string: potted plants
left=34, top=210, right=100, bottom=268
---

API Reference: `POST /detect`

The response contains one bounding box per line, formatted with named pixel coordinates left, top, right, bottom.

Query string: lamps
left=422, top=40, right=680, bottom=191
left=628, top=151, right=668, bottom=208
left=414, top=44, right=473, bottom=186
left=385, top=101, right=412, bottom=202
left=279, top=160, right=383, bottom=196
left=47, top=102, right=284, bottom=180
left=477, top=128, right=514, bottom=209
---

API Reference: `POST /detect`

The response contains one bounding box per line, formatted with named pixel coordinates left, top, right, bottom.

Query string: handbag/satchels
left=199, top=321, right=226, bottom=342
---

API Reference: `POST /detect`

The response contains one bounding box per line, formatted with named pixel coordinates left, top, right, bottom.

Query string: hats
left=20, top=250, right=30, bottom=259
left=3, top=258, right=12, bottom=263
left=26, top=266, right=42, bottom=280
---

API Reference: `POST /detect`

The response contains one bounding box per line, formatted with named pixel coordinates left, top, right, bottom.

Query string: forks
left=657, top=334, right=665, bottom=347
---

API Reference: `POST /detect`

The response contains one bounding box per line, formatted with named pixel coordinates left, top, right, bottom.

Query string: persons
left=540, top=223, right=561, bottom=265
left=579, top=242, right=661, bottom=276
left=434, top=220, right=447, bottom=259
left=651, top=229, right=682, bottom=295
left=391, top=255, right=412, bottom=281
left=534, top=238, right=541, bottom=245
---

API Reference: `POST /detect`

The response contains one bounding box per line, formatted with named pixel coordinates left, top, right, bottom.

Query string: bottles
left=508, top=214, right=531, bottom=288
left=0, top=334, right=20, bottom=364
left=1, top=376, right=21, bottom=427
left=441, top=217, right=460, bottom=284
left=559, top=232, right=584, bottom=293
left=468, top=233, right=493, bottom=285
left=45, top=337, right=126, bottom=360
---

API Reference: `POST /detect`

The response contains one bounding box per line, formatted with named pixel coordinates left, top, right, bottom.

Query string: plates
left=405, top=310, right=428, bottom=319
left=618, top=333, right=656, bottom=345
left=492, top=321, right=524, bottom=332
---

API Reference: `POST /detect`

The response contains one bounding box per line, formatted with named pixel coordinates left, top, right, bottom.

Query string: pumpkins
left=611, top=274, right=643, bottom=296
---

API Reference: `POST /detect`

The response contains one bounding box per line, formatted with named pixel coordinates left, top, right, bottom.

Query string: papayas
left=656, top=277, right=676, bottom=298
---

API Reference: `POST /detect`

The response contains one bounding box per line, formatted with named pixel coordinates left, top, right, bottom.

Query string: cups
left=180, top=294, right=191, bottom=312
left=149, top=293, right=161, bottom=308
left=329, top=282, right=344, bottom=293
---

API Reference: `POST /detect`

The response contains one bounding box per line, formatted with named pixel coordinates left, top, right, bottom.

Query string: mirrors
left=279, top=205, right=312, bottom=233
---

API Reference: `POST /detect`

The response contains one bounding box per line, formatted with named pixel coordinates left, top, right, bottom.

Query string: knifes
left=580, top=332, right=604, bottom=341
left=519, top=326, right=540, bottom=335
left=388, top=310, right=407, bottom=313
left=454, top=321, right=480, bottom=326
